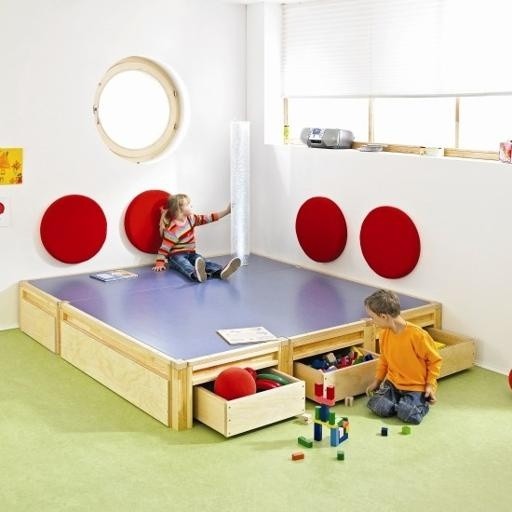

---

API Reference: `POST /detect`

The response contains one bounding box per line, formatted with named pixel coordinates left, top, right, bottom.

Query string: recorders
left=300, top=127, right=353, bottom=149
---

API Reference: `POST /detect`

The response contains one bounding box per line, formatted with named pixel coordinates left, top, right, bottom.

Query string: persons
left=363, top=289, right=445, bottom=425
left=151, top=194, right=242, bottom=283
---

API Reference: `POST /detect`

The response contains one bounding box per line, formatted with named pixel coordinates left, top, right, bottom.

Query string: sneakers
left=193, top=256, right=208, bottom=283
left=218, top=256, right=242, bottom=280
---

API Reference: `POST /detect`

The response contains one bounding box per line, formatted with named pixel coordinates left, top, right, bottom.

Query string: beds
left=19, top=245, right=481, bottom=438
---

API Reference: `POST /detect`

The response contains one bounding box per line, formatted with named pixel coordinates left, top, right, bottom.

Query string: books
left=218, top=327, right=277, bottom=345
left=89, top=270, right=140, bottom=283
